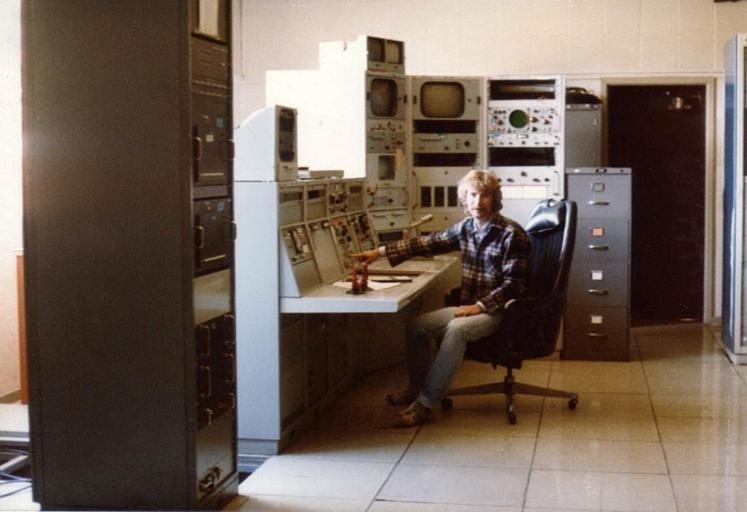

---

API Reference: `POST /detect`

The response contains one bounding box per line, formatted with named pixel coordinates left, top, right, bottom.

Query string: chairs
left=441, top=200, right=579, bottom=422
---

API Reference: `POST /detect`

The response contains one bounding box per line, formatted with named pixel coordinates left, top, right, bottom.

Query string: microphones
left=406, top=214, right=434, bottom=230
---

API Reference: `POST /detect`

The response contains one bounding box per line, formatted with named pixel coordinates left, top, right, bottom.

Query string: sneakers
left=388, top=390, right=423, bottom=405
left=397, top=401, right=430, bottom=426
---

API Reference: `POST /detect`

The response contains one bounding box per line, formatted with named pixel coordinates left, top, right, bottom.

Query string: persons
left=349, top=168, right=531, bottom=428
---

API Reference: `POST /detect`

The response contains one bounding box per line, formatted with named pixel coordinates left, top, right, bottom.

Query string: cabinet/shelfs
left=565, top=168, right=632, bottom=359
left=21, top=0, right=237, bottom=512
left=721, top=34, right=747, bottom=365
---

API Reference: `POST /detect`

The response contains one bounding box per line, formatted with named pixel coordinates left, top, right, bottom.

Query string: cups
left=351, top=264, right=368, bottom=293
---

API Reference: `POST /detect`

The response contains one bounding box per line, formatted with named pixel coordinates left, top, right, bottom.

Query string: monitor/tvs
left=377, top=157, right=391, bottom=180
left=422, top=84, right=464, bottom=117
left=278, top=108, right=294, bottom=162
left=368, top=38, right=384, bottom=62
left=372, top=80, right=397, bottom=115
left=386, top=41, right=401, bottom=64
